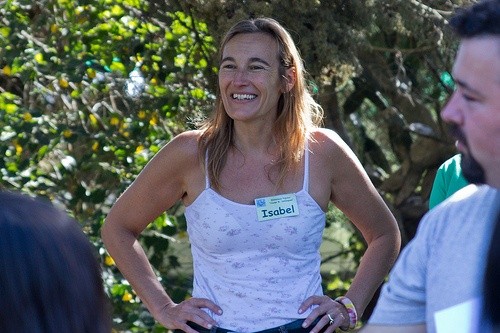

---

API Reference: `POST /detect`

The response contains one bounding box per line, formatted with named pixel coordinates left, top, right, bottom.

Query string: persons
left=101, top=19, right=401, bottom=333
left=362, top=0, right=500, bottom=333
left=0, top=191, right=112, bottom=333
left=429, top=153, right=469, bottom=209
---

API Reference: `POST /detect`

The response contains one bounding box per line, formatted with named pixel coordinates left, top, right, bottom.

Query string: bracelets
left=335, top=295, right=357, bottom=331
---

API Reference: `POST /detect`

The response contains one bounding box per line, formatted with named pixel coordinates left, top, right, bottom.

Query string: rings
left=327, top=314, right=334, bottom=325
